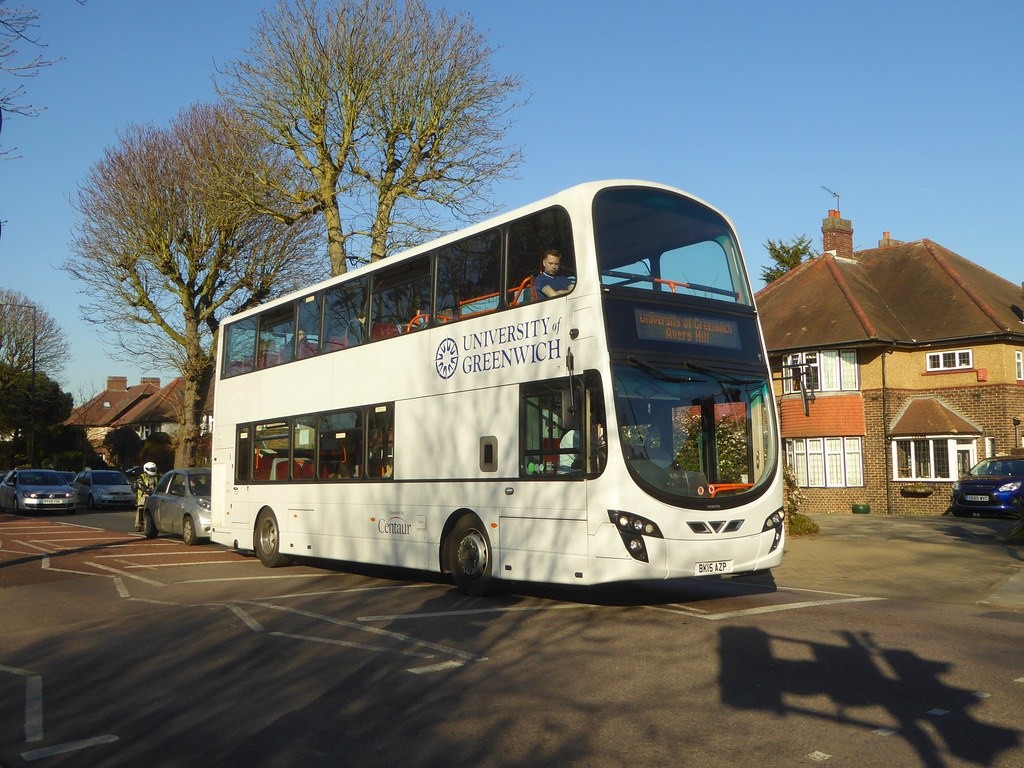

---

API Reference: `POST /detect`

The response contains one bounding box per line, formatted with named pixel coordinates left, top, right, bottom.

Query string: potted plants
left=900, top=484, right=933, bottom=498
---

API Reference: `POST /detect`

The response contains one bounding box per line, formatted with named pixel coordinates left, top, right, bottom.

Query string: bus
left=210, top=179, right=786, bottom=592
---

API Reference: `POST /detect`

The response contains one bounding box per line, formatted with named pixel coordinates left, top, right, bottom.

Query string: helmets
left=143, top=462, right=157, bottom=475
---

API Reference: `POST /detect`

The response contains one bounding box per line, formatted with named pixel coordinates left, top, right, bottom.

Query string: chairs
left=253, top=452, right=362, bottom=480
left=13, top=477, right=23, bottom=486
left=531, top=279, right=544, bottom=303
left=542, top=438, right=560, bottom=471
left=222, top=314, right=419, bottom=379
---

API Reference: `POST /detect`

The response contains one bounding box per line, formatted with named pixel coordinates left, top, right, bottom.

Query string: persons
left=134, top=462, right=159, bottom=532
left=382, top=456, right=394, bottom=478
left=283, top=329, right=312, bottom=360
left=187, top=475, right=203, bottom=496
left=533, top=249, right=575, bottom=301
left=556, top=415, right=605, bottom=476
left=347, top=312, right=370, bottom=346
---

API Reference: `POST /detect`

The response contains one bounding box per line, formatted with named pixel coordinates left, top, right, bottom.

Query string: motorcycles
left=132, top=481, right=152, bottom=530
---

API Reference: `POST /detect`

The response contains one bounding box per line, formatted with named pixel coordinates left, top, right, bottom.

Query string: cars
left=124, top=466, right=141, bottom=478
left=0, top=468, right=79, bottom=515
left=0, top=470, right=8, bottom=484
left=59, top=471, right=77, bottom=484
left=952, top=455, right=1023, bottom=518
left=73, top=470, right=137, bottom=511
left=142, top=465, right=211, bottom=544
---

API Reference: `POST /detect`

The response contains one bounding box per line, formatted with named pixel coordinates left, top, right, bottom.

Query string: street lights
left=1013, top=417, right=1021, bottom=447
left=0, top=301, right=38, bottom=468
